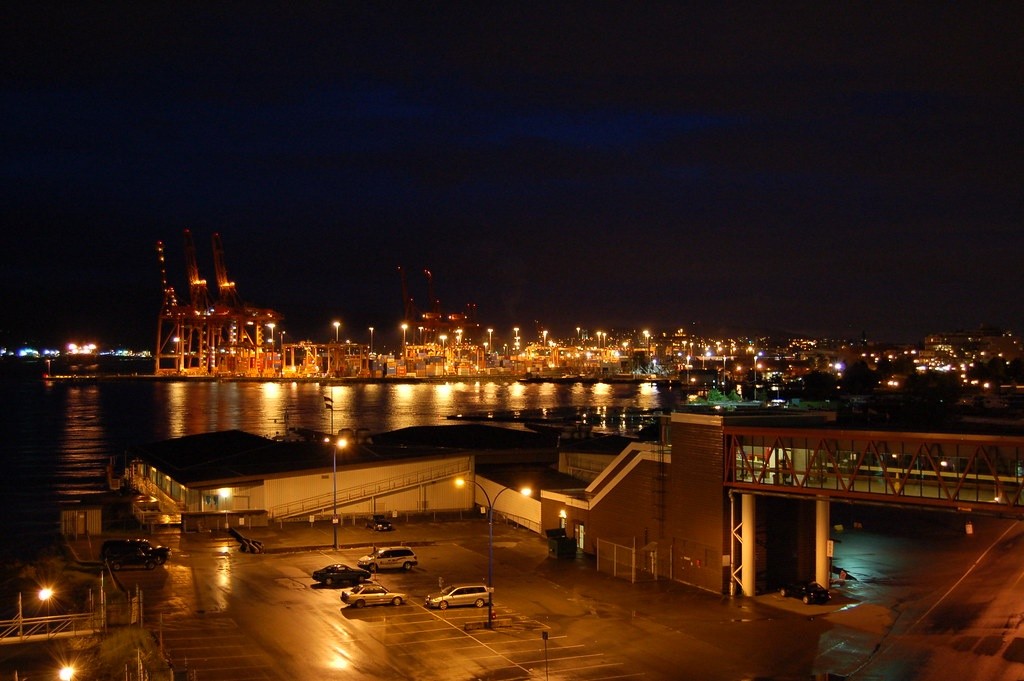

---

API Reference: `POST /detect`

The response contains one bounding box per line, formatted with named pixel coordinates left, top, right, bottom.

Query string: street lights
left=265, top=318, right=655, bottom=351
left=323, top=436, right=348, bottom=549
left=454, top=477, right=531, bottom=628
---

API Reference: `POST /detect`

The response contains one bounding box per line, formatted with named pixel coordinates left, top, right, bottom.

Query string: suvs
left=357, top=546, right=419, bottom=573
left=98, top=538, right=171, bottom=570
left=364, top=513, right=392, bottom=532
left=425, top=583, right=490, bottom=610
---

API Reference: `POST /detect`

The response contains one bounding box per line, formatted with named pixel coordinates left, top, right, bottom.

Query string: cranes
left=154, top=227, right=275, bottom=376
left=396, top=262, right=464, bottom=344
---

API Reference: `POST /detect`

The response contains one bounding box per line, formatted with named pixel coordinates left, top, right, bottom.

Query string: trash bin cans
left=545, top=528, right=578, bottom=559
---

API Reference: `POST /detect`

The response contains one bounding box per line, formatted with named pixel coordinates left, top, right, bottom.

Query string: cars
left=312, top=564, right=371, bottom=585
left=780, top=580, right=831, bottom=604
left=340, top=584, right=409, bottom=608
